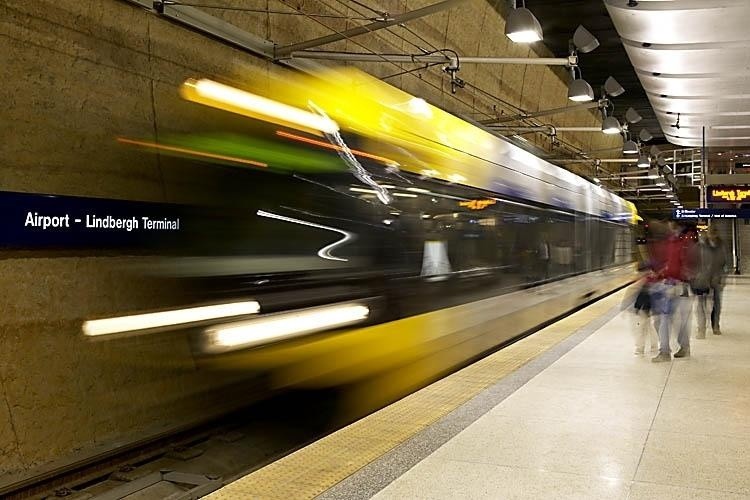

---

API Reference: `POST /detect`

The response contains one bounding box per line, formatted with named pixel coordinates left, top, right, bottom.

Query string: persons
left=533, top=230, right=585, bottom=284
left=627, top=214, right=728, bottom=363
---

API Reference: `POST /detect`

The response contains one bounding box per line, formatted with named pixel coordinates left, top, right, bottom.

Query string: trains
left=175, top=60, right=656, bottom=420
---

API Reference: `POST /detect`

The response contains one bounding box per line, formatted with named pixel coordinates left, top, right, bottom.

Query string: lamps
left=310, top=25, right=600, bottom=105
left=273, top=1, right=543, bottom=55
left=481, top=76, right=627, bottom=139
left=493, top=108, right=684, bottom=214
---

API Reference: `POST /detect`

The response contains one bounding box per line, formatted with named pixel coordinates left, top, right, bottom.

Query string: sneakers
left=652, top=350, right=671, bottom=363
left=712, top=325, right=721, bottom=335
left=673, top=347, right=691, bottom=357
left=697, top=328, right=705, bottom=338
